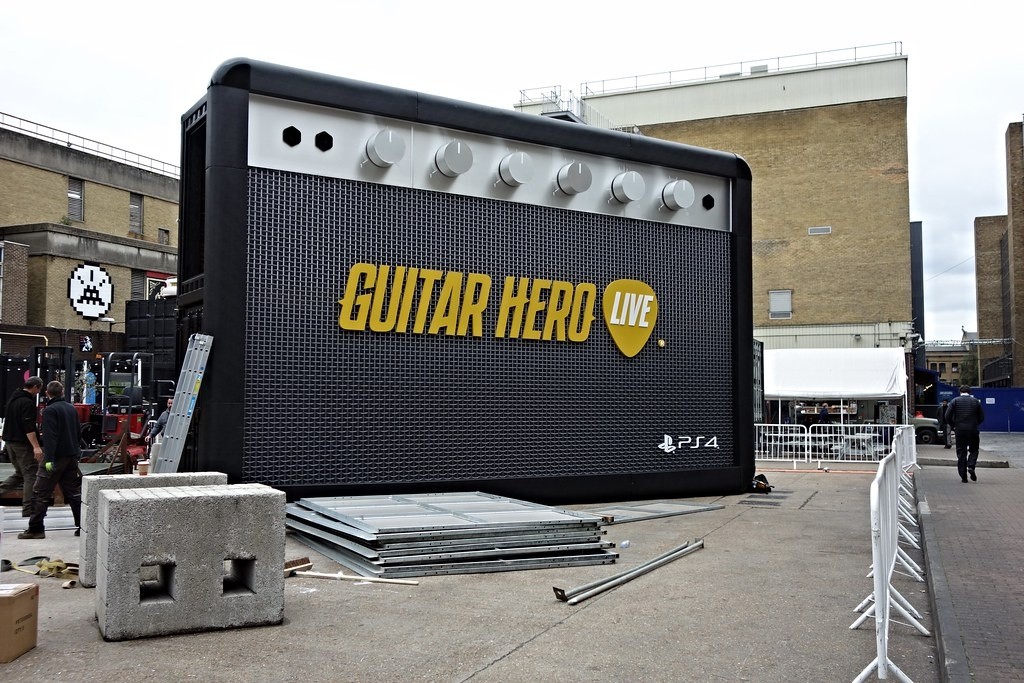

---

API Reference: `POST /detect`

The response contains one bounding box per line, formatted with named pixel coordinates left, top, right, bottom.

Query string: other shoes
left=962, top=479, right=968, bottom=483
left=968, top=467, right=977, bottom=481
left=18, top=530, right=45, bottom=539
left=22, top=512, right=47, bottom=517
left=944, top=445, right=951, bottom=449
left=74, top=527, right=80, bottom=536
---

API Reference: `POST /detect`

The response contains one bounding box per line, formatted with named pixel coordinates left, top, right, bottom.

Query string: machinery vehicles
left=29, top=346, right=101, bottom=449
left=90, top=350, right=175, bottom=462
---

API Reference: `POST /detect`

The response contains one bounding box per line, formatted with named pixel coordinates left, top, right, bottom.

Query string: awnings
left=762, top=348, right=907, bottom=400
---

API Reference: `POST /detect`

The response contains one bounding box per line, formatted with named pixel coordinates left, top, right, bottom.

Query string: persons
left=18, top=381, right=83, bottom=539
left=820, top=403, right=828, bottom=424
left=145, top=397, right=174, bottom=442
left=938, top=399, right=951, bottom=449
left=914, top=411, right=924, bottom=418
left=944, top=386, right=984, bottom=482
left=0, top=376, right=43, bottom=517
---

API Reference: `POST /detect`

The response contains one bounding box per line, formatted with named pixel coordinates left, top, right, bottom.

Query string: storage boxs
left=0, top=583, right=40, bottom=663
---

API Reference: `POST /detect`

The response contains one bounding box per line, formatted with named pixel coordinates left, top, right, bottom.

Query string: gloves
left=45, top=462, right=57, bottom=473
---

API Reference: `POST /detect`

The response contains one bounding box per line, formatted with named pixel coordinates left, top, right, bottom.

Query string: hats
left=960, top=384, right=971, bottom=390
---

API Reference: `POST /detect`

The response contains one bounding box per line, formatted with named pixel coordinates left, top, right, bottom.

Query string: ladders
left=150, top=333, right=214, bottom=473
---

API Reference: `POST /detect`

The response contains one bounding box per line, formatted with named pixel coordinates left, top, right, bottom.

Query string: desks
left=836, top=436, right=878, bottom=461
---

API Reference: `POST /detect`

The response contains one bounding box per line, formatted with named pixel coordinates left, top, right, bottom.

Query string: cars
left=863, top=413, right=940, bottom=443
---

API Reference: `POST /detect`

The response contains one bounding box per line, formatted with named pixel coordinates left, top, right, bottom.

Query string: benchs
left=831, top=443, right=888, bottom=461
